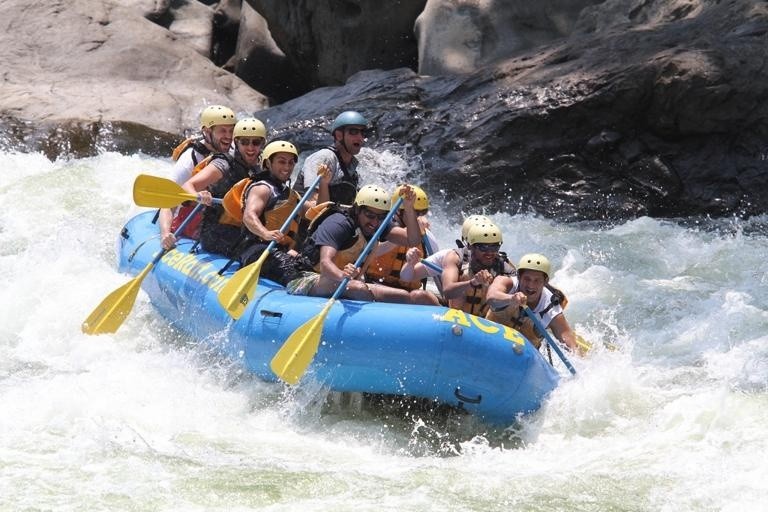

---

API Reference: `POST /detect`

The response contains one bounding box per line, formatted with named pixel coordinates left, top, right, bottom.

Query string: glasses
left=347, top=127, right=369, bottom=138
left=477, top=244, right=500, bottom=251
left=364, top=210, right=387, bottom=219
left=415, top=209, right=428, bottom=216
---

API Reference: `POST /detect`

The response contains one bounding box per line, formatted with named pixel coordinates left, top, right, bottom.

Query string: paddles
left=218, top=169, right=325, bottom=332
left=269, top=192, right=410, bottom=387
left=81, top=199, right=212, bottom=337
left=420, top=259, right=600, bottom=358
left=133, top=171, right=224, bottom=214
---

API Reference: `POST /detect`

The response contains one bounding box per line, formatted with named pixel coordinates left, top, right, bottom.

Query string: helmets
left=462, top=215, right=502, bottom=245
left=331, top=111, right=367, bottom=134
left=201, top=105, right=298, bottom=160
left=354, top=183, right=429, bottom=211
left=518, top=254, right=550, bottom=277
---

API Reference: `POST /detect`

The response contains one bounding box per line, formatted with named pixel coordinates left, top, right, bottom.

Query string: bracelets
left=469, top=279, right=481, bottom=289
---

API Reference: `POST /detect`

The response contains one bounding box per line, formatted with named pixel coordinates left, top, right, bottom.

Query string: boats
left=113, top=196, right=564, bottom=418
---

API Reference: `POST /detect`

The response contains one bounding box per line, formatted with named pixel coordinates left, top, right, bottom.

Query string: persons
left=241, top=139, right=334, bottom=286
left=293, top=109, right=370, bottom=254
left=440, top=221, right=518, bottom=320
left=181, top=116, right=265, bottom=253
left=400, top=213, right=493, bottom=308
left=362, top=182, right=444, bottom=293
left=157, top=104, right=235, bottom=252
left=485, top=251, right=583, bottom=358
left=286, top=183, right=421, bottom=303
